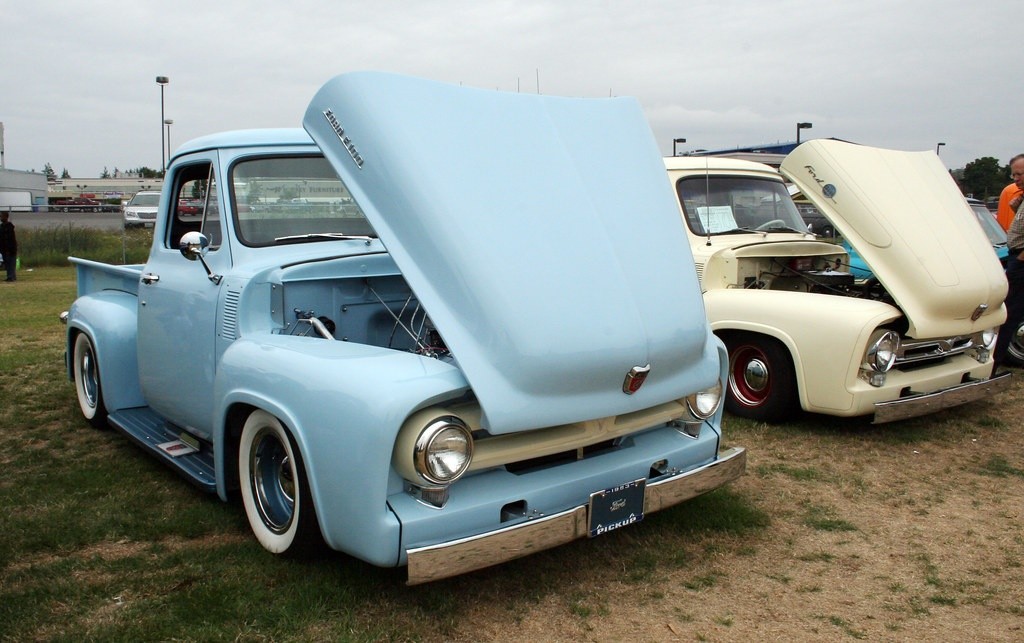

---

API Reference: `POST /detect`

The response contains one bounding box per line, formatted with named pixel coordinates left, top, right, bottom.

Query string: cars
left=122, top=190, right=161, bottom=230
left=291, top=197, right=308, bottom=204
left=662, top=137, right=1012, bottom=426
left=178, top=199, right=198, bottom=216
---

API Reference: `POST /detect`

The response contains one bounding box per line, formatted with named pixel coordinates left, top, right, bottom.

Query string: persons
left=0, top=211, right=18, bottom=282
left=993, top=147, right=1024, bottom=361
left=996, top=182, right=1023, bottom=232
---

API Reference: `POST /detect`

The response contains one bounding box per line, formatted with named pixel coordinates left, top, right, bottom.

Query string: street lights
left=673, top=138, right=686, bottom=157
left=155, top=76, right=169, bottom=181
left=936, top=142, right=946, bottom=155
left=797, top=123, right=813, bottom=145
left=164, top=118, right=173, bottom=162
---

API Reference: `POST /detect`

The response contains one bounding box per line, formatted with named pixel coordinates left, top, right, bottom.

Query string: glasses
left=1010, top=172, right=1024, bottom=178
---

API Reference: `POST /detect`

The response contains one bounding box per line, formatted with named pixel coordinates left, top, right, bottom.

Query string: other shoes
left=4, top=278, right=14, bottom=282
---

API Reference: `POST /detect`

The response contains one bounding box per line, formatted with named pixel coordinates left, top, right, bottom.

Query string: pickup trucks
left=56, top=195, right=101, bottom=213
left=60, top=71, right=747, bottom=589
